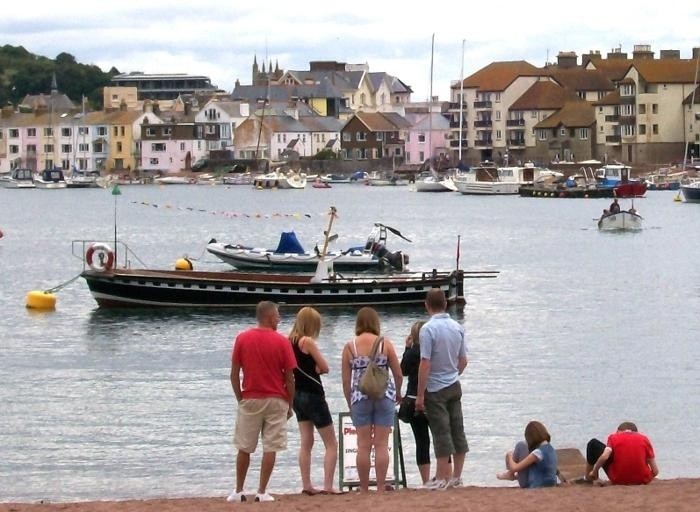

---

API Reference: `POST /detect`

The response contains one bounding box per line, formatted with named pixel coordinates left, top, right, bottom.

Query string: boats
left=1, top=166, right=35, bottom=190
left=63, top=236, right=506, bottom=320
left=451, top=160, right=700, bottom=206
left=200, top=223, right=412, bottom=276
left=112, top=172, right=356, bottom=189
left=366, top=176, right=410, bottom=187
left=593, top=210, right=644, bottom=230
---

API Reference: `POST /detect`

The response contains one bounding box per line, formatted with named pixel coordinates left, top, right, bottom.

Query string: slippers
left=356, top=486, right=370, bottom=496
left=375, top=486, right=395, bottom=495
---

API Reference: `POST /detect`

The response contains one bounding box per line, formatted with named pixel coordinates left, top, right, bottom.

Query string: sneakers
left=415, top=483, right=427, bottom=490
left=496, top=469, right=518, bottom=480
left=226, top=488, right=247, bottom=503
left=426, top=479, right=447, bottom=492
left=254, top=492, right=275, bottom=502
left=569, top=474, right=592, bottom=488
left=449, top=478, right=465, bottom=488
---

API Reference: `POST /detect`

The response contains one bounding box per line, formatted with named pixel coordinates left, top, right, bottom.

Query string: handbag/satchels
left=357, top=360, right=389, bottom=401
left=397, top=396, right=417, bottom=423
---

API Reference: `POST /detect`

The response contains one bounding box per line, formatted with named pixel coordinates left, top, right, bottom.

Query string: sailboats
left=34, top=90, right=110, bottom=191
left=412, top=33, right=496, bottom=194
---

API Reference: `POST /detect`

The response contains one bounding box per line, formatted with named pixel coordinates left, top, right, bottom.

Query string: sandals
left=302, top=487, right=321, bottom=496
left=321, top=489, right=342, bottom=496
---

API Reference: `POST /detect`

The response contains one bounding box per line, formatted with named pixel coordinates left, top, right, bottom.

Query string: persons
left=497, top=421, right=558, bottom=489
left=284, top=307, right=347, bottom=495
left=600, top=199, right=636, bottom=219
left=225, top=299, right=296, bottom=501
left=341, top=305, right=403, bottom=491
left=570, top=422, right=658, bottom=486
left=415, top=288, right=469, bottom=489
left=401, top=321, right=455, bottom=488
